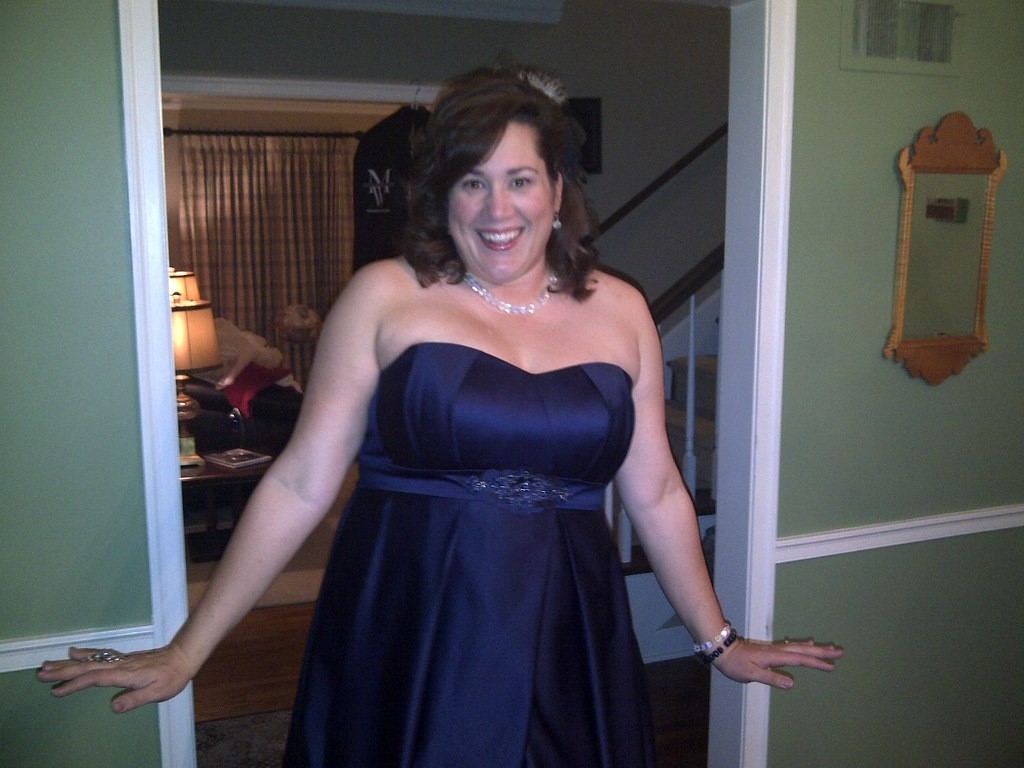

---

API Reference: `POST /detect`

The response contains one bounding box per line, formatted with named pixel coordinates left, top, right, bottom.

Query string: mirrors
left=881, top=108, right=1009, bottom=388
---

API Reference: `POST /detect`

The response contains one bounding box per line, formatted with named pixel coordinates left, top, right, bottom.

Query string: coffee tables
left=180, top=449, right=274, bottom=564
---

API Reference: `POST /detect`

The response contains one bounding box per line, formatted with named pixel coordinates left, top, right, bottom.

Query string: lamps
left=166, top=265, right=224, bottom=469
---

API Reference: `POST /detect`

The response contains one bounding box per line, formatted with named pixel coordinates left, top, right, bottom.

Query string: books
left=203, top=447, right=272, bottom=469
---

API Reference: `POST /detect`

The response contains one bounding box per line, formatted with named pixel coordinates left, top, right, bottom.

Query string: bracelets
left=691, top=620, right=732, bottom=654
left=702, top=629, right=737, bottom=665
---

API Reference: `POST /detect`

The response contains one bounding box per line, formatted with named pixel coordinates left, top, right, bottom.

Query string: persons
left=37, top=67, right=846, bottom=767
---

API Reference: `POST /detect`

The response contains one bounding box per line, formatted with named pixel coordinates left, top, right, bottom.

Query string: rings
left=84, top=650, right=122, bottom=663
left=784, top=638, right=790, bottom=646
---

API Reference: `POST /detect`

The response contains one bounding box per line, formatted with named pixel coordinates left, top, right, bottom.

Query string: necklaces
left=446, top=268, right=559, bottom=315
